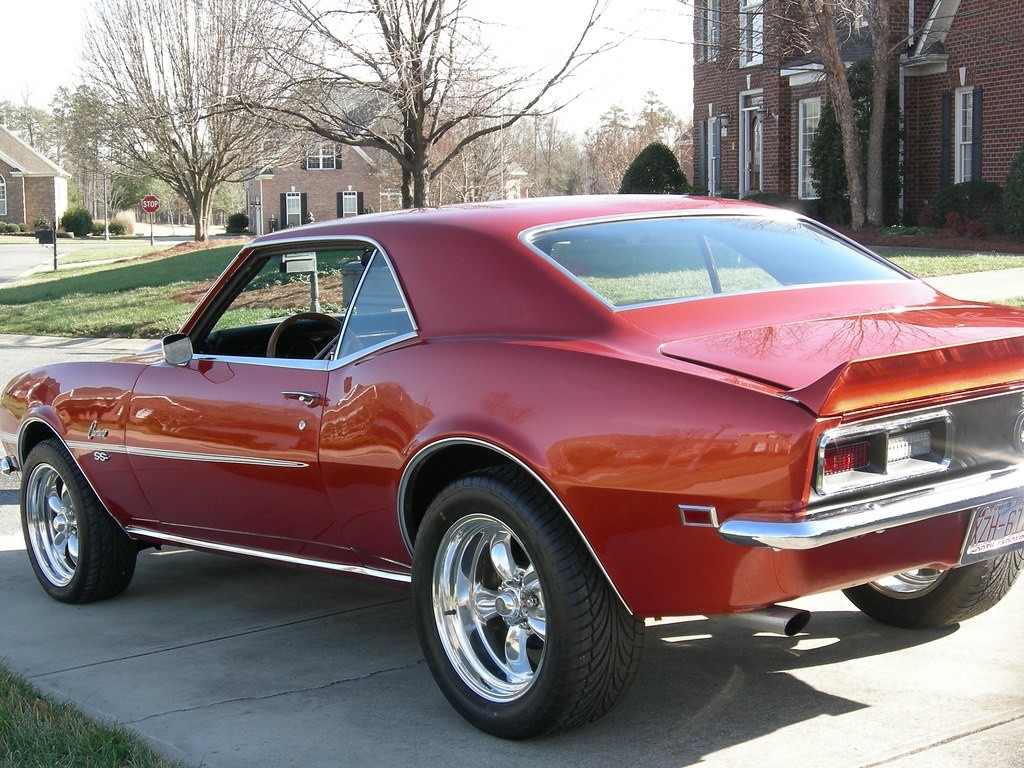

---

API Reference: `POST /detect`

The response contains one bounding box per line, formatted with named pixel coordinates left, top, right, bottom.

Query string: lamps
left=757, top=100, right=770, bottom=123
left=719, top=112, right=732, bottom=128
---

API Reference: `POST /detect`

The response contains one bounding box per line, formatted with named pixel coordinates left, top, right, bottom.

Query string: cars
left=0, top=192, right=1024, bottom=743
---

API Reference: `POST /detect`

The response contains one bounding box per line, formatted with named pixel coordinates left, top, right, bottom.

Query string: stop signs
left=140, top=195, right=160, bottom=213
